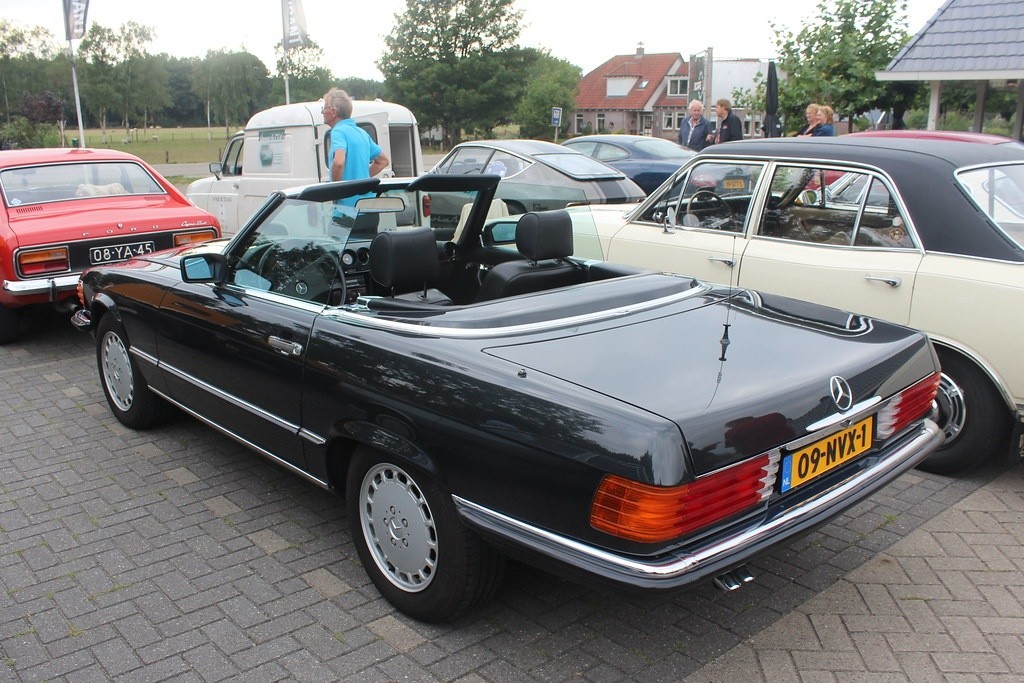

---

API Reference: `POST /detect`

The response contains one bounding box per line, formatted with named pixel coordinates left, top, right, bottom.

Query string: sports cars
left=77, top=172, right=949, bottom=621
left=423, top=140, right=646, bottom=233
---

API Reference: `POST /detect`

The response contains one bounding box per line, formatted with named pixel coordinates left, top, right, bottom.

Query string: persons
left=320, top=88, right=391, bottom=241
left=706, top=98, right=743, bottom=146
left=798, top=106, right=835, bottom=137
left=792, top=103, right=820, bottom=137
left=678, top=98, right=714, bottom=152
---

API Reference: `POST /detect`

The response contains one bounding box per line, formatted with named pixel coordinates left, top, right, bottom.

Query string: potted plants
left=566, top=126, right=574, bottom=138
left=583, top=122, right=592, bottom=136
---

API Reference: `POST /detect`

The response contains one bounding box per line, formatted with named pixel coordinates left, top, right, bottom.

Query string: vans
left=186, top=98, right=433, bottom=252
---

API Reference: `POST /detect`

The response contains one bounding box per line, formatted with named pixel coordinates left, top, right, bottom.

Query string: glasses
left=321, top=105, right=331, bottom=111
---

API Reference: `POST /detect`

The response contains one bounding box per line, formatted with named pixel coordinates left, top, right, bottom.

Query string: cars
left=560, top=133, right=756, bottom=205
left=478, top=130, right=1024, bottom=474
left=0, top=146, right=224, bottom=347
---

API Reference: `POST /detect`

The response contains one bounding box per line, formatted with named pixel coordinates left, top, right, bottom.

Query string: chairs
left=74, top=183, right=126, bottom=197
left=479, top=210, right=583, bottom=300
left=494, top=158, right=520, bottom=177
left=368, top=227, right=455, bottom=306
